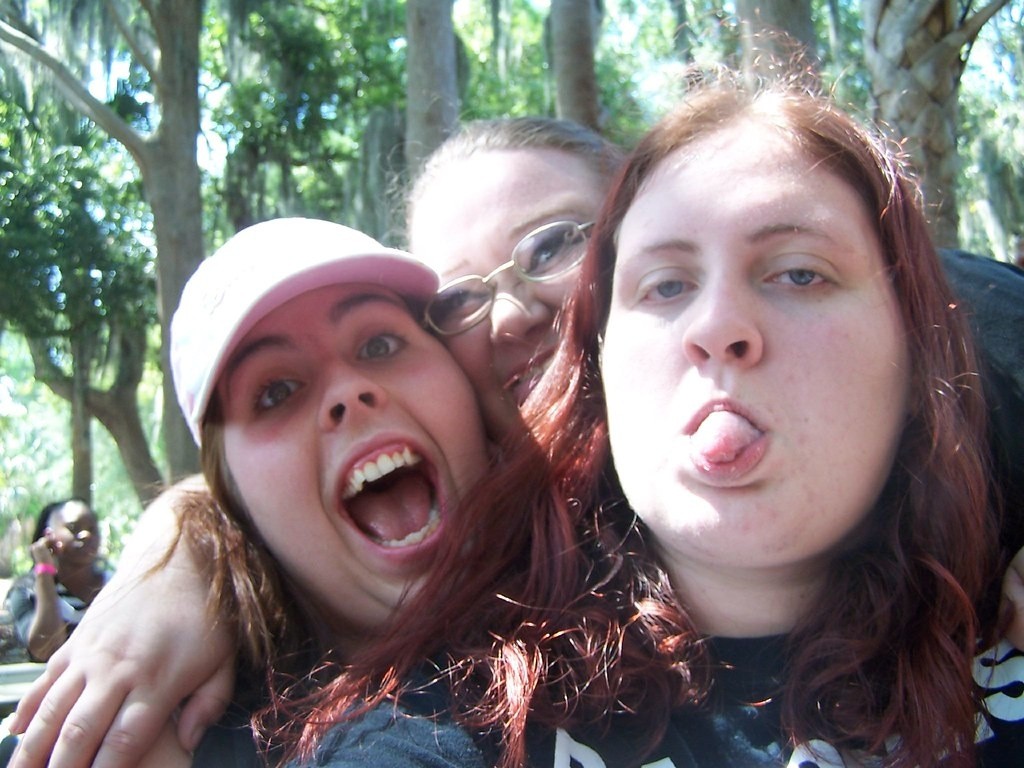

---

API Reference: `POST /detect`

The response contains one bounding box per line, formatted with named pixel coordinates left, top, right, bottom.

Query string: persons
left=240, top=23, right=1024, bottom=768
left=0, top=117, right=1024, bottom=768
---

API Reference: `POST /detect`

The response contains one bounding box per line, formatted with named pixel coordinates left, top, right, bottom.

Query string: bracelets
left=34, top=562, right=56, bottom=576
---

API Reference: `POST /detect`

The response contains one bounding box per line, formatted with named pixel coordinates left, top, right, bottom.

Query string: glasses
left=420, top=218, right=595, bottom=337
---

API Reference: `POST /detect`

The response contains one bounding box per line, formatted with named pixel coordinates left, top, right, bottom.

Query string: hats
left=168, top=217, right=442, bottom=450
